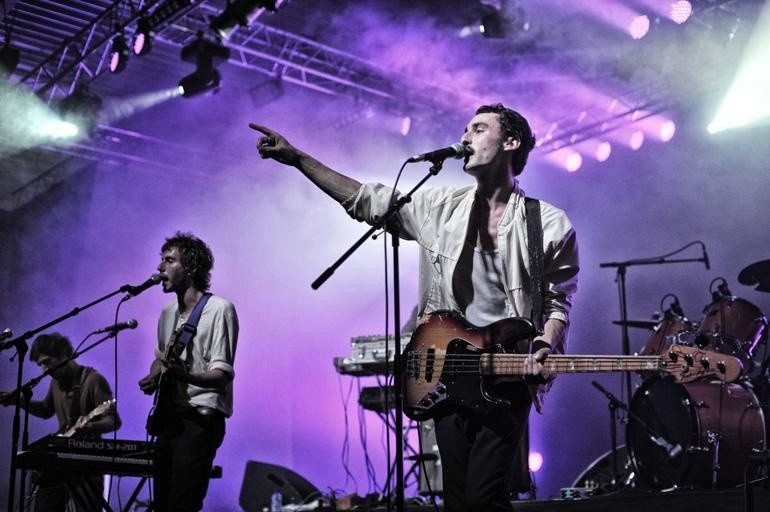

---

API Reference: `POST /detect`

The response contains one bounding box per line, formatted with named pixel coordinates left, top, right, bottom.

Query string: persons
left=137, top=228, right=240, bottom=512
left=0, top=332, right=122, bottom=512
left=248, top=102, right=579, bottom=512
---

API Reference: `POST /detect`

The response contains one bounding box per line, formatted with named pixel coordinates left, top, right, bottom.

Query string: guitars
left=399, top=309, right=743, bottom=423
left=56, top=398, right=115, bottom=437
left=146, top=347, right=190, bottom=437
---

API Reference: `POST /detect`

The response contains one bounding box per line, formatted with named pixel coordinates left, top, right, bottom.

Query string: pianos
left=13, top=434, right=154, bottom=477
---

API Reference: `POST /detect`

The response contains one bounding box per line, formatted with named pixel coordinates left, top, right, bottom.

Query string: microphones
left=122, top=273, right=162, bottom=301
left=410, top=144, right=469, bottom=164
left=702, top=250, right=711, bottom=270
left=94, top=318, right=139, bottom=335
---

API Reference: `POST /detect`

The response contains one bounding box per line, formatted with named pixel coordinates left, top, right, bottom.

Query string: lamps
left=109, top=1, right=284, bottom=98
left=477, top=1, right=541, bottom=41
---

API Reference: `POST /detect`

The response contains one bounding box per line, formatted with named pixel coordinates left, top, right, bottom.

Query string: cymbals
left=738, top=259, right=769, bottom=293
left=611, top=319, right=658, bottom=329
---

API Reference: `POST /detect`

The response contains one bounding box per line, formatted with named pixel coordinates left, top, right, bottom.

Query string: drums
left=625, top=373, right=768, bottom=489
left=694, top=296, right=766, bottom=373
left=634, top=315, right=699, bottom=379
